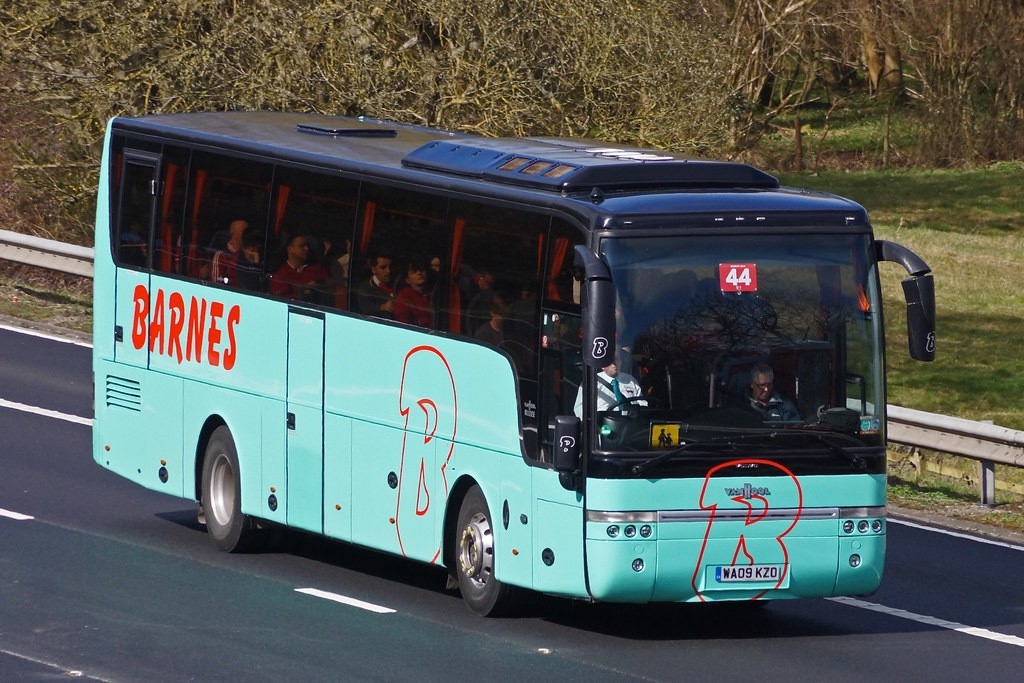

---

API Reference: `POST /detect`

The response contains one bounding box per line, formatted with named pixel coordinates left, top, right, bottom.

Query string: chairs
left=120, top=209, right=851, bottom=430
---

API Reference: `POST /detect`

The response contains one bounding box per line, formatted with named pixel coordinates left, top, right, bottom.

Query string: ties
left=611, top=380, right=628, bottom=409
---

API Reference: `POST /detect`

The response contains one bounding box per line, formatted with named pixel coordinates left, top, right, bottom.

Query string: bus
left=91, top=110, right=937, bottom=616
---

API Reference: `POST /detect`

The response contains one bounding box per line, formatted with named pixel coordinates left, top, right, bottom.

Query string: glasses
left=754, top=383, right=773, bottom=390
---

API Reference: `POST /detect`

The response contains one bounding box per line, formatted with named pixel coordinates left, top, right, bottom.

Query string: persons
left=212, top=220, right=275, bottom=289
left=466, top=274, right=519, bottom=347
left=272, top=236, right=329, bottom=295
left=748, top=365, right=799, bottom=422
left=356, top=254, right=403, bottom=315
left=573, top=354, right=648, bottom=420
left=392, top=255, right=440, bottom=328
